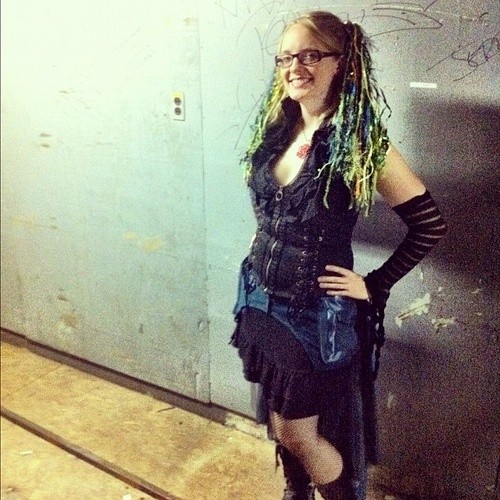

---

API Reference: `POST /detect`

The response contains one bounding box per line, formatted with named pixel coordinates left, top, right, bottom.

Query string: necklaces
left=293, top=116, right=316, bottom=160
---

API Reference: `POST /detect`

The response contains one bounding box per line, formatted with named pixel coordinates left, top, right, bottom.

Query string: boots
left=307, top=467, right=352, bottom=500
left=276, top=443, right=312, bottom=499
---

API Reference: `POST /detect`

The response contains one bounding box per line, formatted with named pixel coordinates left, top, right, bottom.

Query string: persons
left=227, top=10, right=447, bottom=500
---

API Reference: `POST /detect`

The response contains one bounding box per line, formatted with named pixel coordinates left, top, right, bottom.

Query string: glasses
left=275, top=49, right=348, bottom=68
